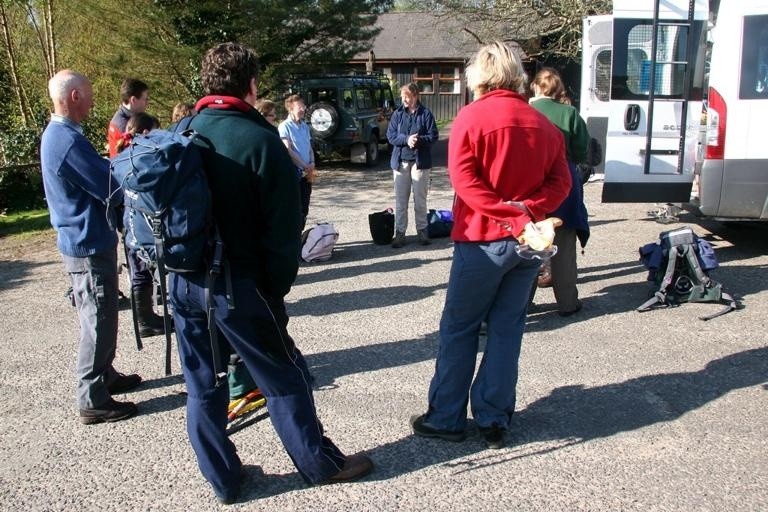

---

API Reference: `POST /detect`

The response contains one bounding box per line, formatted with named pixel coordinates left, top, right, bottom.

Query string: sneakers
left=100, top=364, right=141, bottom=395
left=476, top=423, right=504, bottom=450
left=526, top=303, right=537, bottom=315
left=416, top=230, right=432, bottom=245
left=478, top=321, right=487, bottom=336
left=392, top=231, right=407, bottom=248
left=559, top=299, right=582, bottom=317
left=77, top=397, right=137, bottom=425
left=410, top=413, right=465, bottom=442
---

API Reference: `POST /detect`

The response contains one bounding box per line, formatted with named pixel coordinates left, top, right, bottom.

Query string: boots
left=130, top=291, right=175, bottom=338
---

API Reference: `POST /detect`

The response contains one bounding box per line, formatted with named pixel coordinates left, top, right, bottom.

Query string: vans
left=580, top=0, right=768, bottom=224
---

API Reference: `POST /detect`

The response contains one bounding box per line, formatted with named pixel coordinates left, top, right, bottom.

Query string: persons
left=109, top=67, right=315, bottom=339
left=410, top=40, right=572, bottom=449
left=537, top=74, right=602, bottom=288
left=528, top=68, right=590, bottom=317
left=167, top=41, right=373, bottom=505
left=385, top=84, right=439, bottom=248
left=40, top=68, right=143, bottom=425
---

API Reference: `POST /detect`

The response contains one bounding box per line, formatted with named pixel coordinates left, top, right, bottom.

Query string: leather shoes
left=305, top=451, right=372, bottom=483
left=537, top=264, right=558, bottom=287
left=216, top=454, right=243, bottom=505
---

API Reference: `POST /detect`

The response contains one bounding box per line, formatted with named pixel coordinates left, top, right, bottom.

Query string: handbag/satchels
left=226, top=351, right=258, bottom=399
left=426, top=208, right=454, bottom=239
left=368, top=208, right=395, bottom=245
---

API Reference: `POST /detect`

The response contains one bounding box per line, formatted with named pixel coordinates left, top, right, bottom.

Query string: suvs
left=293, top=70, right=399, bottom=167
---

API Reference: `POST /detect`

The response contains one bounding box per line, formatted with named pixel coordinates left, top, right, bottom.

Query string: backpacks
left=63, top=285, right=127, bottom=307
left=112, top=123, right=220, bottom=274
left=638, top=225, right=724, bottom=304
left=301, top=222, right=339, bottom=262
